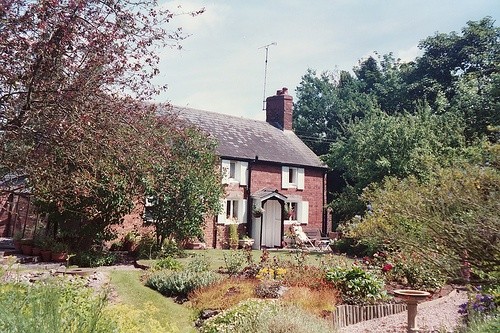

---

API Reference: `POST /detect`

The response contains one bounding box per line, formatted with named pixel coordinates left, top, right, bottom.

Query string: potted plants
left=111, top=243, right=118, bottom=251
left=239, top=236, right=255, bottom=246
left=228, top=224, right=239, bottom=249
left=252, top=207, right=265, bottom=218
left=13, top=231, right=68, bottom=262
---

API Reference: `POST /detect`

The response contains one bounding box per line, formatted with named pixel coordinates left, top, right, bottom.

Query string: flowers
left=284, top=210, right=295, bottom=217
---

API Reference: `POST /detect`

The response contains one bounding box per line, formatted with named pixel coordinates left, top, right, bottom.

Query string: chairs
left=292, top=226, right=320, bottom=252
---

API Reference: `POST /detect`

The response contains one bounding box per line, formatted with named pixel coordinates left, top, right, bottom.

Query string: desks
left=316, top=240, right=330, bottom=251
